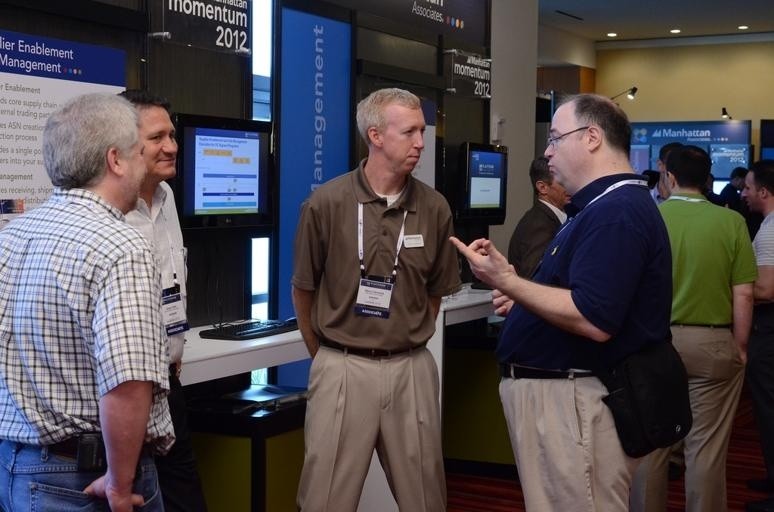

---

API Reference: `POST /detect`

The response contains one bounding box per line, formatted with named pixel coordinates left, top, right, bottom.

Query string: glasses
left=546, top=127, right=590, bottom=150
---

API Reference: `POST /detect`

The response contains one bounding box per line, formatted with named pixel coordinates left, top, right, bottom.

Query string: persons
left=719, top=167, right=751, bottom=237
left=742, top=160, right=773, bottom=511
left=630, top=146, right=759, bottom=512
left=641, top=170, right=660, bottom=191
left=124, top=99, right=208, bottom=512
left=509, top=156, right=573, bottom=281
left=293, top=87, right=462, bottom=511
left=702, top=174, right=719, bottom=205
left=648, top=142, right=686, bottom=207
left=1, top=91, right=176, bottom=512
left=448, top=95, right=693, bottom=511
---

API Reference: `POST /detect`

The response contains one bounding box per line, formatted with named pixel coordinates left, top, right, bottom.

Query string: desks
left=177, top=316, right=309, bottom=387
left=426, top=282, right=495, bottom=434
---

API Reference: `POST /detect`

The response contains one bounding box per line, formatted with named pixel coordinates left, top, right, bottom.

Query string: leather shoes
left=746, top=478, right=774, bottom=493
left=745, top=496, right=774, bottom=512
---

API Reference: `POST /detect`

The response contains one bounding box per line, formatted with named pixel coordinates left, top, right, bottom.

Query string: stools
left=208, top=386, right=309, bottom=512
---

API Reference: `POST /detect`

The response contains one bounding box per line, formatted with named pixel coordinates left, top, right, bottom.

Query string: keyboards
left=193, top=319, right=296, bottom=342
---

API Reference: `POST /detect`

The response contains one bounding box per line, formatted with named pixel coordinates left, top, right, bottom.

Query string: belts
left=48, top=437, right=156, bottom=461
left=501, top=363, right=596, bottom=381
left=670, top=322, right=731, bottom=328
left=320, top=339, right=426, bottom=360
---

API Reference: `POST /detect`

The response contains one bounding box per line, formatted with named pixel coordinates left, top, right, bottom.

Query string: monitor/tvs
left=760, top=118, right=774, bottom=174
left=167, top=113, right=281, bottom=242
left=463, top=141, right=509, bottom=228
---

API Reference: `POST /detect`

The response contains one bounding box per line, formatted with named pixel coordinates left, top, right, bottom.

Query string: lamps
left=721, top=107, right=731, bottom=121
left=608, top=87, right=638, bottom=100
